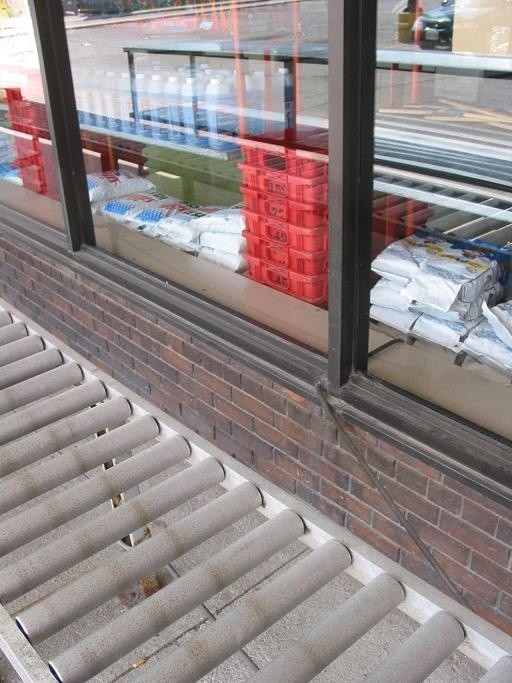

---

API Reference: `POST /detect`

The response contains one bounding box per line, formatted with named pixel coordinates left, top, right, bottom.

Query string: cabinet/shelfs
left=120, top=42, right=512, bottom=193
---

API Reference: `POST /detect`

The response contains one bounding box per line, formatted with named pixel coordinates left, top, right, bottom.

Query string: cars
left=411, top=0, right=512, bottom=51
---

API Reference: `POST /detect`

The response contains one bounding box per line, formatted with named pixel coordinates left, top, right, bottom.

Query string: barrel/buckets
left=70, top=55, right=293, bottom=151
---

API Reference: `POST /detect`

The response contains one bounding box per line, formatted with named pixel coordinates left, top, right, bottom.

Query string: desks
left=0, top=88, right=512, bottom=256
